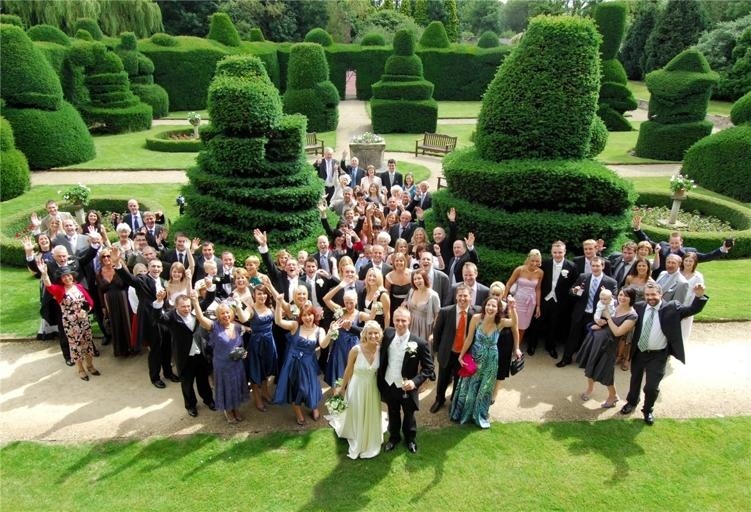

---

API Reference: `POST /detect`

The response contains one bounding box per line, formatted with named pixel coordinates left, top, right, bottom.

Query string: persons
left=353, top=184, right=360, bottom=197
left=429, top=208, right=456, bottom=272
left=358, top=245, right=394, bottom=280
left=253, top=228, right=312, bottom=305
left=419, top=252, right=450, bottom=353
left=381, top=159, right=403, bottom=198
left=416, top=181, right=432, bottom=210
left=679, top=252, right=704, bottom=348
left=329, top=164, right=350, bottom=201
left=146, top=225, right=169, bottom=255
left=19, top=225, right=99, bottom=366
left=382, top=214, right=397, bottom=230
left=164, top=238, right=195, bottom=307
left=166, top=230, right=191, bottom=269
left=596, top=238, right=638, bottom=289
left=656, top=254, right=688, bottom=305
left=233, top=284, right=277, bottom=411
left=621, top=281, right=710, bottom=424
left=362, top=267, right=390, bottom=330
left=143, top=246, right=170, bottom=281
left=329, top=264, right=367, bottom=312
left=576, top=286, right=639, bottom=407
left=273, top=250, right=291, bottom=272
left=361, top=165, right=382, bottom=192
left=322, top=320, right=388, bottom=459
left=109, top=246, right=180, bottom=388
left=313, top=147, right=339, bottom=207
left=402, top=269, right=441, bottom=344
left=228, top=267, right=256, bottom=312
left=42, top=217, right=64, bottom=240
left=311, top=234, right=339, bottom=276
left=111, top=223, right=135, bottom=265
left=430, top=284, right=482, bottom=414
left=135, top=211, right=165, bottom=239
left=448, top=232, right=479, bottom=284
left=360, top=218, right=370, bottom=244
left=274, top=293, right=345, bottom=424
left=528, top=240, right=580, bottom=359
left=329, top=228, right=353, bottom=260
left=449, top=295, right=518, bottom=429
left=614, top=259, right=654, bottom=371
left=189, top=289, right=248, bottom=423
left=196, top=279, right=220, bottom=310
left=245, top=256, right=271, bottom=294
left=218, top=251, right=239, bottom=292
left=316, top=201, right=365, bottom=246
left=344, top=208, right=357, bottom=229
left=376, top=232, right=395, bottom=253
left=322, top=278, right=378, bottom=397
left=587, top=289, right=616, bottom=330
left=383, top=197, right=401, bottom=218
left=386, top=238, right=413, bottom=268
left=407, top=227, right=431, bottom=259
left=297, top=250, right=309, bottom=277
left=571, top=239, right=612, bottom=276
left=40, top=200, right=78, bottom=232
left=128, top=263, right=149, bottom=354
left=503, top=249, right=544, bottom=351
left=555, top=256, right=618, bottom=367
left=125, top=232, right=167, bottom=265
left=444, top=261, right=490, bottom=306
left=261, top=273, right=308, bottom=320
left=152, top=288, right=218, bottom=417
left=401, top=192, right=417, bottom=219
left=403, top=171, right=417, bottom=199
left=195, top=260, right=229, bottom=304
left=341, top=149, right=365, bottom=188
left=339, top=255, right=353, bottom=281
left=409, top=244, right=445, bottom=270
left=631, top=215, right=735, bottom=278
left=192, top=238, right=223, bottom=283
left=329, top=187, right=355, bottom=215
left=355, top=244, right=374, bottom=273
left=364, top=183, right=383, bottom=204
left=299, top=257, right=344, bottom=373
left=36, top=256, right=101, bottom=382
left=33, top=233, right=58, bottom=340
left=388, top=207, right=425, bottom=248
left=389, top=185, right=402, bottom=202
left=79, top=209, right=111, bottom=289
left=489, top=281, right=521, bottom=404
left=378, top=306, right=435, bottom=453
left=96, top=249, right=135, bottom=357
left=112, top=199, right=165, bottom=239
left=636, top=240, right=662, bottom=272
left=30, top=212, right=91, bottom=289
left=385, top=253, right=412, bottom=328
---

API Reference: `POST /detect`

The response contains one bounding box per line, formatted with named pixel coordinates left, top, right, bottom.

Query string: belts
left=643, top=347, right=668, bottom=353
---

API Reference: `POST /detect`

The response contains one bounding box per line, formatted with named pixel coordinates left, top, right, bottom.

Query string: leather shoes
left=557, top=356, right=573, bottom=367
left=153, top=379, right=166, bottom=389
left=202, top=398, right=216, bottom=411
left=406, top=439, right=418, bottom=454
left=273, top=376, right=279, bottom=384
left=644, top=412, right=654, bottom=424
left=383, top=437, right=395, bottom=452
left=101, top=336, right=110, bottom=345
left=430, top=400, right=445, bottom=413
left=526, top=343, right=536, bottom=357
left=621, top=402, right=633, bottom=414
left=549, top=347, right=558, bottom=359
left=188, top=405, right=198, bottom=417
left=429, top=371, right=437, bottom=381
left=166, top=374, right=180, bottom=382
left=66, top=359, right=74, bottom=366
left=316, top=370, right=322, bottom=375
left=92, top=350, right=100, bottom=357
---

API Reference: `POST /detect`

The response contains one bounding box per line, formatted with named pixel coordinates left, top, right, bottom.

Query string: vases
left=194, top=121, right=198, bottom=123
left=72, top=204, right=85, bottom=211
left=673, top=189, right=686, bottom=198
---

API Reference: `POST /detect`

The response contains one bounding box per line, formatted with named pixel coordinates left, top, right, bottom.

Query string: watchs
left=360, top=213, right=365, bottom=218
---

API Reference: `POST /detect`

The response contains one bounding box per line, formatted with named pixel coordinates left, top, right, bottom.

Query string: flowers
left=187, top=112, right=201, bottom=124
left=57, top=182, right=92, bottom=209
left=671, top=175, right=692, bottom=192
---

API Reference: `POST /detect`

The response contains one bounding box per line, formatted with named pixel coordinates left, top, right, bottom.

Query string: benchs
left=304, top=132, right=325, bottom=159
left=415, top=131, right=458, bottom=157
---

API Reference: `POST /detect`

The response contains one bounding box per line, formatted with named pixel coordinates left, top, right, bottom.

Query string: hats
left=54, top=265, right=79, bottom=281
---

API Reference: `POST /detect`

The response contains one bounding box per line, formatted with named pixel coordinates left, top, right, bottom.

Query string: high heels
left=490, top=388, right=497, bottom=405
left=614, top=355, right=624, bottom=366
left=254, top=400, right=267, bottom=412
left=311, top=409, right=321, bottom=422
left=210, top=382, right=215, bottom=389
left=294, top=413, right=306, bottom=426
left=622, top=358, right=630, bottom=371
left=261, top=392, right=276, bottom=405
left=223, top=408, right=238, bottom=424
left=78, top=369, right=89, bottom=382
left=601, top=398, right=620, bottom=407
left=581, top=392, right=590, bottom=402
left=171, top=357, right=176, bottom=366
left=87, top=366, right=101, bottom=376
left=232, top=409, right=244, bottom=422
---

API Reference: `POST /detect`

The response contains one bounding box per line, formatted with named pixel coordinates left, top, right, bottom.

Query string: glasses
left=102, top=254, right=112, bottom=258
left=55, top=255, right=68, bottom=259
left=286, top=263, right=298, bottom=267
left=63, top=224, right=75, bottom=229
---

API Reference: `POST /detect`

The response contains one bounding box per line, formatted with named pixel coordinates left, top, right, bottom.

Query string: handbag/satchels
left=457, top=353, right=477, bottom=378
left=510, top=352, right=525, bottom=377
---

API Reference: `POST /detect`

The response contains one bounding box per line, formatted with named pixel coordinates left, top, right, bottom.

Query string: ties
left=179, top=253, right=184, bottom=264
left=401, top=227, right=406, bottom=237
left=321, top=255, right=326, bottom=270
left=586, top=278, right=598, bottom=311
left=450, top=258, right=458, bottom=283
left=419, top=194, right=424, bottom=208
left=390, top=173, right=394, bottom=184
left=134, top=215, right=139, bottom=232
left=149, top=230, right=153, bottom=235
left=455, top=311, right=465, bottom=352
left=351, top=167, right=355, bottom=188
left=658, top=275, right=670, bottom=287
left=615, top=262, right=629, bottom=284
left=327, top=160, right=332, bottom=185
left=349, top=287, right=354, bottom=290
left=637, top=308, right=656, bottom=353
